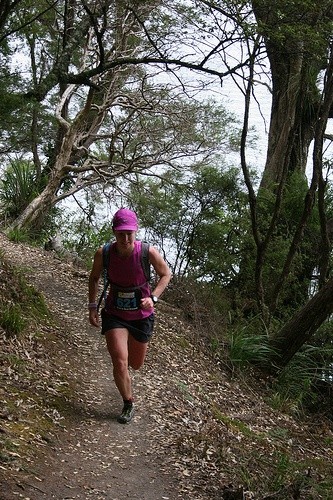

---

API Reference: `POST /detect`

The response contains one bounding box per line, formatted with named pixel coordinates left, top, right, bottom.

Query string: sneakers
left=117, top=404, right=134, bottom=423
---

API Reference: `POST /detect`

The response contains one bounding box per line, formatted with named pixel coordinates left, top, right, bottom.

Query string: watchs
left=149, top=295, right=158, bottom=304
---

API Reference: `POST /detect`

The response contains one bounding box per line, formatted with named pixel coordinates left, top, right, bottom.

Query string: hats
left=112, top=208, right=139, bottom=231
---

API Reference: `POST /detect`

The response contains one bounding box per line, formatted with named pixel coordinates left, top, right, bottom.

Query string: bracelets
left=88, top=303, right=97, bottom=309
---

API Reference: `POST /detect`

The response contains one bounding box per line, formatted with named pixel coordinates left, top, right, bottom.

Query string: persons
left=88, top=209, right=171, bottom=424
